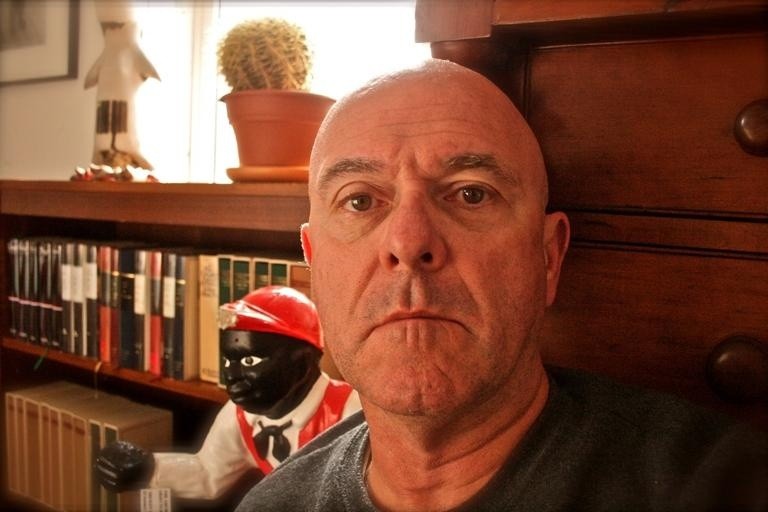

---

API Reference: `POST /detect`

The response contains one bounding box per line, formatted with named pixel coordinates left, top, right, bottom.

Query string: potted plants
left=215, top=11, right=335, bottom=169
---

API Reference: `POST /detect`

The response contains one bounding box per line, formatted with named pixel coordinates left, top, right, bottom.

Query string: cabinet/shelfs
left=1, top=179, right=314, bottom=510
left=414, top=0, right=767, bottom=407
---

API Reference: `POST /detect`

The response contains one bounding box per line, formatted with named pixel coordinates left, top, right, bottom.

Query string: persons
left=237, top=60, right=768, bottom=512
left=96, top=286, right=364, bottom=500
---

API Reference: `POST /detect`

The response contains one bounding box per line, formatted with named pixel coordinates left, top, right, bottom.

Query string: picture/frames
left=0, top=0, right=78, bottom=86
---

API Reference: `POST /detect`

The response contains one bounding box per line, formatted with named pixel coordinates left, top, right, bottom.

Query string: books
left=4, top=381, right=173, bottom=512
left=1, top=239, right=310, bottom=389
